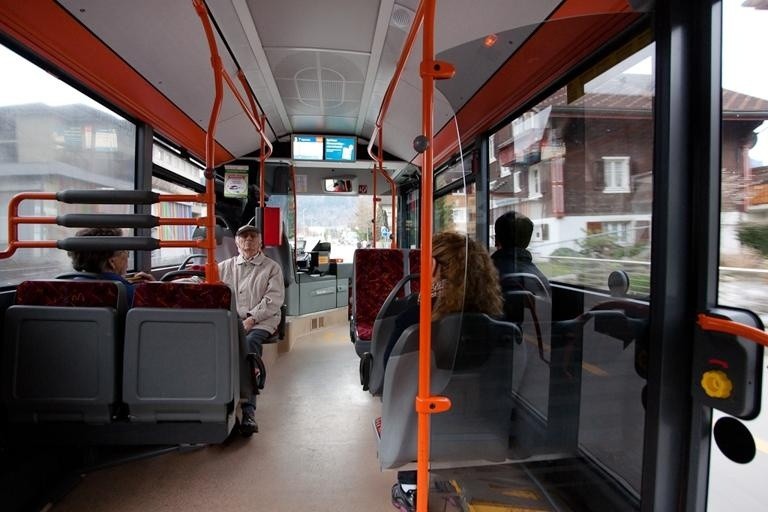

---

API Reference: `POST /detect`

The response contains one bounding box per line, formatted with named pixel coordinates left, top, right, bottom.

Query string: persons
left=68, top=229, right=157, bottom=309
left=333, top=183, right=345, bottom=192
left=490, top=211, right=552, bottom=296
left=383, top=232, right=505, bottom=512
left=217, top=225, right=286, bottom=438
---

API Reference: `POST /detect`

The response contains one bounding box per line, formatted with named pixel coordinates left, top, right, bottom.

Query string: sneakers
left=391, top=483, right=416, bottom=512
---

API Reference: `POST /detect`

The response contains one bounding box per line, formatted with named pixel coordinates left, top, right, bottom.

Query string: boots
left=241, top=415, right=258, bottom=434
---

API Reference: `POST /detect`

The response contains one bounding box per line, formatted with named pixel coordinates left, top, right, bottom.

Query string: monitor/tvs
left=324, top=135, right=358, bottom=162
left=291, top=134, right=324, bottom=161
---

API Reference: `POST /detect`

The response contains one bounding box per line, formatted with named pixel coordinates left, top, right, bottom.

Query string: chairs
left=346, top=247, right=649, bottom=475
left=8, top=280, right=240, bottom=447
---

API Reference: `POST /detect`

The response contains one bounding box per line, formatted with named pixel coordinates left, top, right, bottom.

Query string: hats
left=235, top=225, right=259, bottom=236
